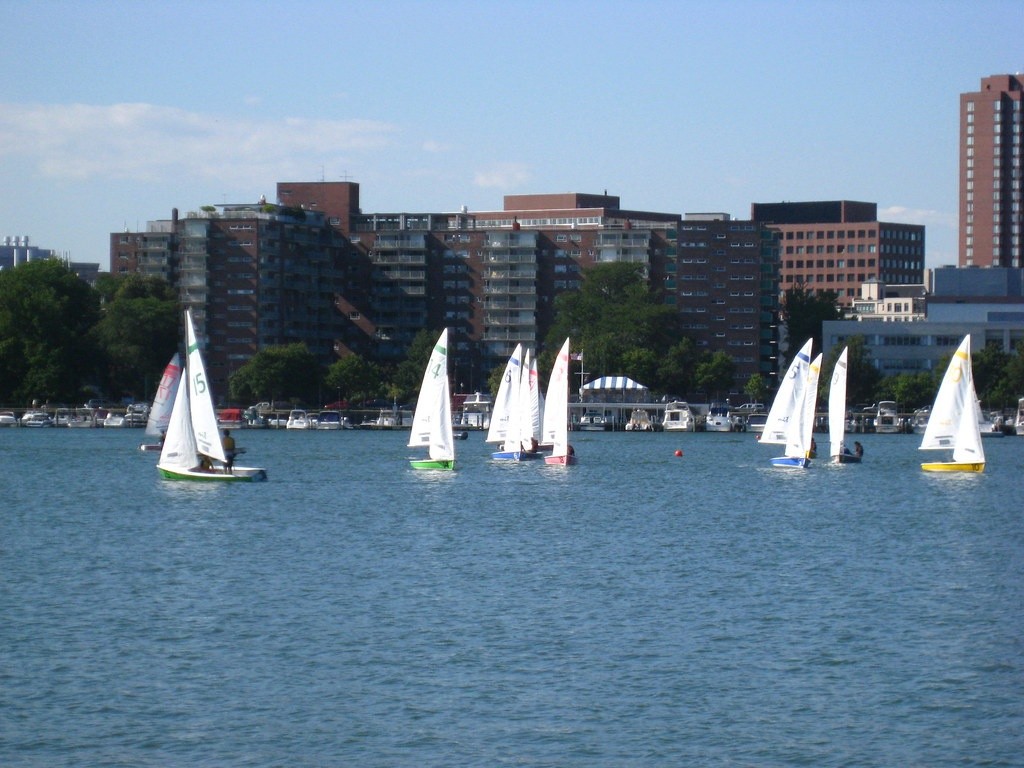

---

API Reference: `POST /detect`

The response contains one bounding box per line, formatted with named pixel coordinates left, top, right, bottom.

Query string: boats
left=0, top=398, right=1024, bottom=437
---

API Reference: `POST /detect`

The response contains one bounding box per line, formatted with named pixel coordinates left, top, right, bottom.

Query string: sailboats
left=918, top=334, right=987, bottom=473
left=406, top=328, right=458, bottom=471
left=829, top=345, right=861, bottom=464
left=485, top=337, right=577, bottom=466
left=758, top=337, right=823, bottom=470
left=139, top=309, right=267, bottom=483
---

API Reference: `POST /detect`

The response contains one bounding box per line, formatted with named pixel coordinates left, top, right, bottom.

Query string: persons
left=843, top=444, right=853, bottom=455
left=220, top=429, right=235, bottom=474
left=854, top=442, right=863, bottom=456
left=198, top=454, right=214, bottom=474
left=811, top=437, right=817, bottom=458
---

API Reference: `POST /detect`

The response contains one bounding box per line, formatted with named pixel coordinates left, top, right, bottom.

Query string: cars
left=913, top=404, right=932, bottom=414
left=863, top=403, right=878, bottom=414
left=399, top=400, right=417, bottom=410
left=324, top=400, right=350, bottom=410
left=249, top=402, right=271, bottom=410
left=88, top=399, right=119, bottom=408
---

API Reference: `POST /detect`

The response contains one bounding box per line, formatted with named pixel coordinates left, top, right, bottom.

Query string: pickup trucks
left=733, top=403, right=764, bottom=412
